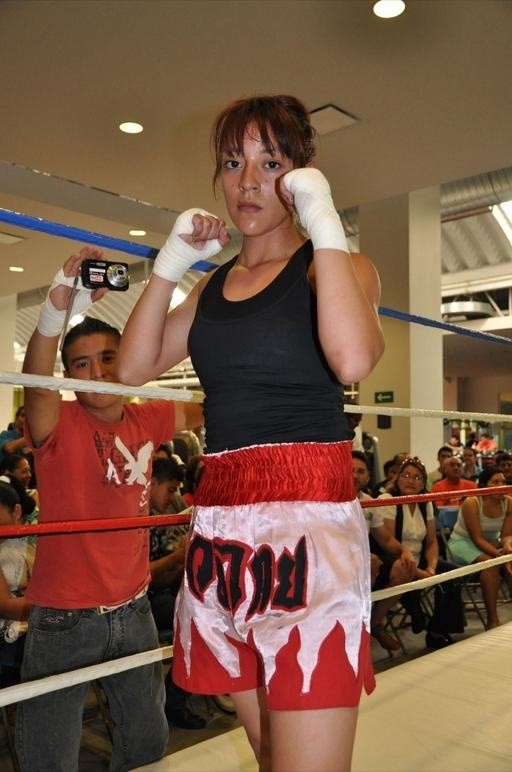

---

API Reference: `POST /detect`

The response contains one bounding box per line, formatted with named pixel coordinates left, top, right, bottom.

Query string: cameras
left=81, top=259, right=130, bottom=292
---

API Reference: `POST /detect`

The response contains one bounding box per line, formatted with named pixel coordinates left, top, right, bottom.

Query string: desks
left=455, top=453, right=493, bottom=470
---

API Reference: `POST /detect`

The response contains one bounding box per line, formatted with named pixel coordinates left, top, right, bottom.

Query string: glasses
left=401, top=473, right=422, bottom=480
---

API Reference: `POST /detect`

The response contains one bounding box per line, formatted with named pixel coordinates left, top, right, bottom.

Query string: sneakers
left=212, top=694, right=236, bottom=711
left=372, top=625, right=399, bottom=650
left=166, top=705, right=205, bottom=729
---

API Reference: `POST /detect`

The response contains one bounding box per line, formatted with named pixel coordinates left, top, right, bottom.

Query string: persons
left=353, top=431, right=511, bottom=649
left=116, top=96, right=385, bottom=771
left=1, top=247, right=203, bottom=771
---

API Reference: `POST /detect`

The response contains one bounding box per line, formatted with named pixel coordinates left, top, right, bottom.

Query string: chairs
left=1, top=677, right=114, bottom=771
left=377, top=640, right=394, bottom=656
left=436, top=508, right=512, bottom=629
left=382, top=573, right=455, bottom=655
left=91, top=648, right=213, bottom=747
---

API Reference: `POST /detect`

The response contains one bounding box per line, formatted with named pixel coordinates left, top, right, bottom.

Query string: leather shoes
left=411, top=612, right=453, bottom=647
